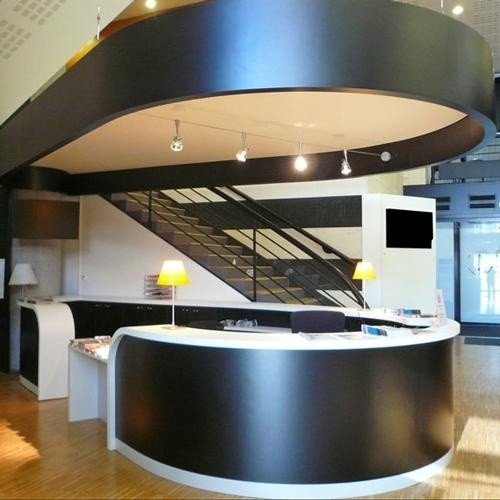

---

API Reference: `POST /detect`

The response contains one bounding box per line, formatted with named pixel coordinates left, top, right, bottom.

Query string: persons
left=487, top=302, right=495, bottom=315
left=484, top=265, right=497, bottom=301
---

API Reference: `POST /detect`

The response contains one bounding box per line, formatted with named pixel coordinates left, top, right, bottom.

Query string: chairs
left=289, top=310, right=347, bottom=333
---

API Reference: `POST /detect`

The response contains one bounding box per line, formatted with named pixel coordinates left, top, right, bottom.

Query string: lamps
left=155, top=258, right=191, bottom=331
left=169, top=119, right=352, bottom=177
left=350, top=257, right=379, bottom=314
left=7, top=262, right=38, bottom=304
